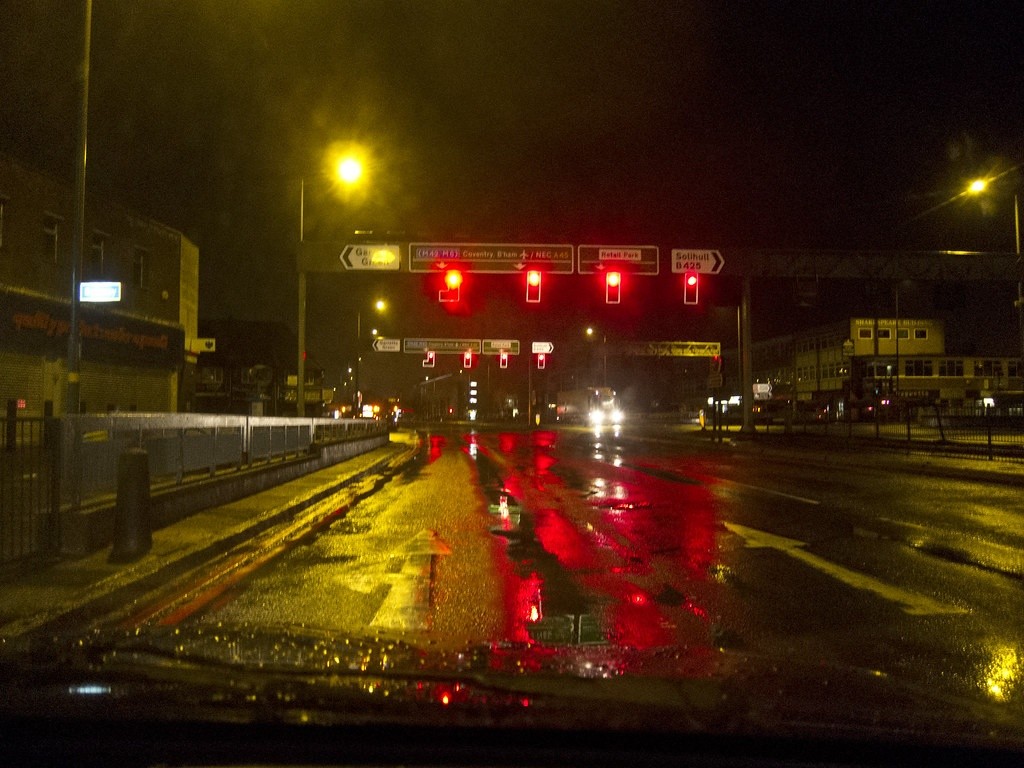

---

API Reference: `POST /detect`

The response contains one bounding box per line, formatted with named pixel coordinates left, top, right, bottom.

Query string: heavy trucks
left=555, top=387, right=621, bottom=425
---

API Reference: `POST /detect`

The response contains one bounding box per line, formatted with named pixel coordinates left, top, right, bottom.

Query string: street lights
left=294, top=154, right=362, bottom=418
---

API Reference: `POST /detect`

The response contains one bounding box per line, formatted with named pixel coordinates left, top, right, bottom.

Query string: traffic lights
left=438, top=268, right=462, bottom=303
left=500, top=352, right=508, bottom=369
left=525, top=270, right=542, bottom=304
left=463, top=352, right=472, bottom=368
left=538, top=353, right=545, bottom=370
left=606, top=271, right=621, bottom=304
left=684, top=271, right=699, bottom=306
left=422, top=351, right=436, bottom=369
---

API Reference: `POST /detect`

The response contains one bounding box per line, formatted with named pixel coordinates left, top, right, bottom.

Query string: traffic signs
left=371, top=338, right=401, bottom=353
left=482, top=339, right=520, bottom=356
left=671, top=248, right=725, bottom=275
left=532, top=342, right=554, bottom=354
left=577, top=244, right=660, bottom=276
left=338, top=244, right=401, bottom=271
left=402, top=337, right=481, bottom=355
left=408, top=242, right=574, bottom=275
